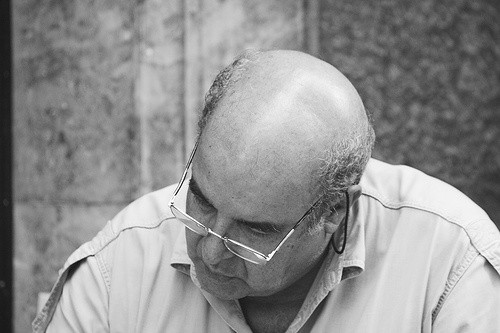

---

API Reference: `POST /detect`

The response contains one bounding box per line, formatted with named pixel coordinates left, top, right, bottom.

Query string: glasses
left=169, top=132, right=329, bottom=266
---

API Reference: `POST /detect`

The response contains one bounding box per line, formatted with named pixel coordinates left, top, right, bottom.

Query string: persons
left=31, top=49, right=500, bottom=333
